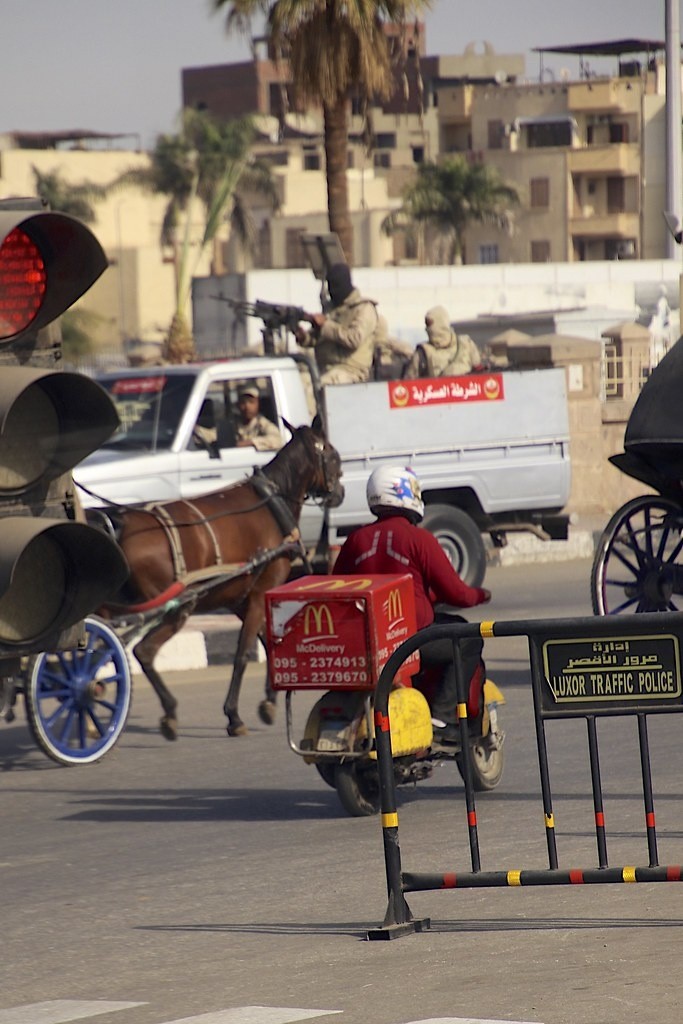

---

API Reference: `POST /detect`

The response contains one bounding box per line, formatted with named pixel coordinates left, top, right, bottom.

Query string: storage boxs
left=264, top=573, right=420, bottom=692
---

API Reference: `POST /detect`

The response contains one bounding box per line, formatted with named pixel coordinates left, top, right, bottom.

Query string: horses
left=82, top=411, right=347, bottom=742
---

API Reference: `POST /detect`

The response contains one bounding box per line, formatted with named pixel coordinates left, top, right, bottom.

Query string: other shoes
left=431, top=721, right=459, bottom=742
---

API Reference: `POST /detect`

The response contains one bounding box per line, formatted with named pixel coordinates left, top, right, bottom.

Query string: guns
left=206, top=291, right=317, bottom=339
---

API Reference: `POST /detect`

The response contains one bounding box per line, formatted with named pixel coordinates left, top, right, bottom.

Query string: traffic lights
left=0, top=196, right=128, bottom=663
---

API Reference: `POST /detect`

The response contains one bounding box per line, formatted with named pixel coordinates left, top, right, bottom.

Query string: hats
left=238, top=384, right=260, bottom=399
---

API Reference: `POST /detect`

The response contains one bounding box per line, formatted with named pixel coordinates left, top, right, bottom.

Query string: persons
left=371, top=316, right=412, bottom=379
left=294, top=266, right=379, bottom=389
left=215, top=385, right=283, bottom=452
left=404, top=306, right=482, bottom=378
left=328, top=464, right=492, bottom=744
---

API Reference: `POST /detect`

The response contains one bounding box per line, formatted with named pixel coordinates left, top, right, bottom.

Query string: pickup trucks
left=74, top=350, right=569, bottom=612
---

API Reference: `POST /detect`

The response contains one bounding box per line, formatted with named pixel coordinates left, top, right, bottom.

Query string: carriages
left=1, top=413, right=346, bottom=764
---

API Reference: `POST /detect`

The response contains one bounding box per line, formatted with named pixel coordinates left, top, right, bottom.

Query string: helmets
left=366, top=465, right=425, bottom=523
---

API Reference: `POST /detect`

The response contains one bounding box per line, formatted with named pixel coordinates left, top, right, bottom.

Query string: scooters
left=267, top=573, right=506, bottom=816
